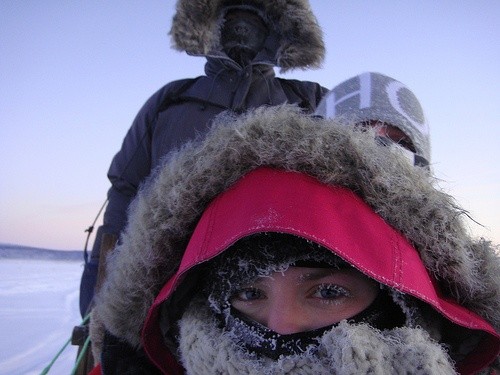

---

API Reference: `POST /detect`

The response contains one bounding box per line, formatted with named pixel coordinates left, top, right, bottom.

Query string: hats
left=313, top=73, right=430, bottom=162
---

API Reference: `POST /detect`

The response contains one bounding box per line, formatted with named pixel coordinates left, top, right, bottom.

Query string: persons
left=309, top=71, right=430, bottom=180
left=88, top=101, right=500, bottom=375
left=72, top=1, right=327, bottom=374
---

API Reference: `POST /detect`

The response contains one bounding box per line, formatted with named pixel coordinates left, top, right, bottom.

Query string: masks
left=218, top=290, right=407, bottom=363
